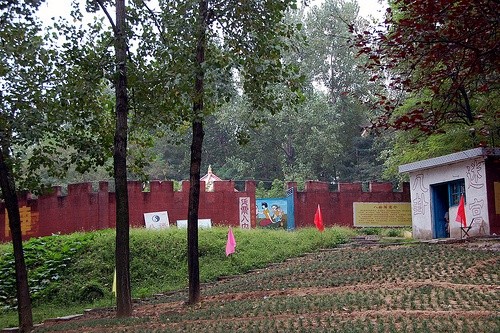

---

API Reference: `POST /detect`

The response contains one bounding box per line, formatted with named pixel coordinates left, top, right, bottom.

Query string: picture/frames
left=143, top=210, right=169, bottom=229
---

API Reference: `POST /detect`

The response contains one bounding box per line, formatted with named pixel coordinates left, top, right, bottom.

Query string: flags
left=312, top=205, right=325, bottom=232
left=224, top=227, right=237, bottom=257
left=455, top=195, right=468, bottom=228
